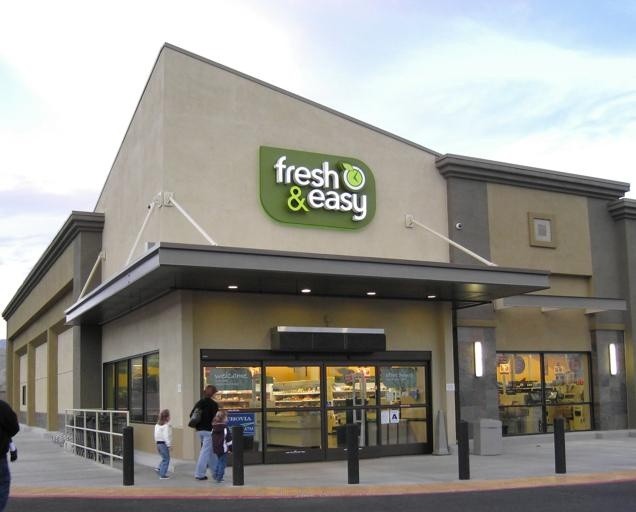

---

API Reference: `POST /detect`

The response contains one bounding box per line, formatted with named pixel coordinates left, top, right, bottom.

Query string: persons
left=154, top=407, right=175, bottom=479
left=189, top=384, right=219, bottom=480
left=209, top=410, right=233, bottom=483
left=0, top=400, right=20, bottom=511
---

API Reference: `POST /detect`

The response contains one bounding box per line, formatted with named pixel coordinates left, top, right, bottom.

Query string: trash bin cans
left=71, top=416, right=126, bottom=463
left=475, top=418, right=503, bottom=455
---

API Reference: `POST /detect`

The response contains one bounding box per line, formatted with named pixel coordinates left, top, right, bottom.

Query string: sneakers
left=153, top=467, right=209, bottom=481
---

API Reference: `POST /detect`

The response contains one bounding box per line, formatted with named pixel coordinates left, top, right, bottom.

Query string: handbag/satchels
left=187, top=407, right=204, bottom=429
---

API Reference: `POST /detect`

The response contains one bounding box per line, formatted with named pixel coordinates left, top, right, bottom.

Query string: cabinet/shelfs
left=268, top=383, right=390, bottom=434
